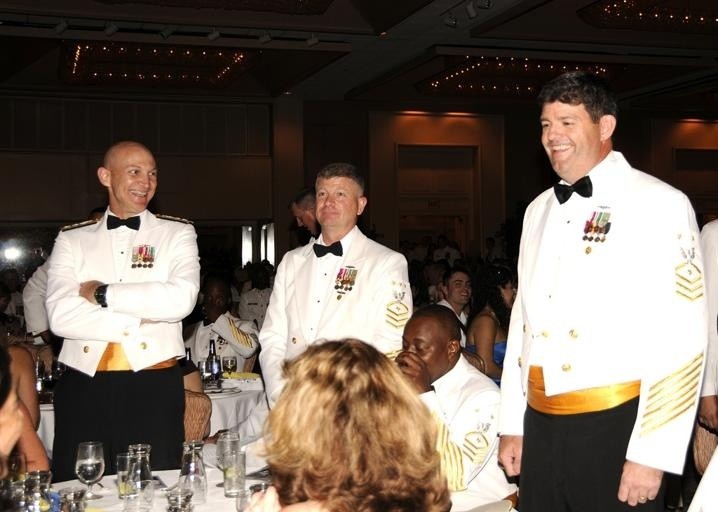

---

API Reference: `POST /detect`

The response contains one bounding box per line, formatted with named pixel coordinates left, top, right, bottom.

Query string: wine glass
left=215, top=431, right=241, bottom=487
left=75, top=441, right=105, bottom=500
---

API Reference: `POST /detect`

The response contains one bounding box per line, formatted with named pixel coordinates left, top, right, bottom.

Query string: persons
left=498, top=70, right=709, bottom=512
left=0, top=183, right=717, bottom=512
left=44, top=141, right=202, bottom=484
left=258, top=163, right=415, bottom=414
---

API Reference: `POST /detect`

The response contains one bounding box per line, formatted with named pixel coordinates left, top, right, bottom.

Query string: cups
left=74, top=496, right=87, bottom=512
left=117, top=452, right=141, bottom=500
left=0, top=452, right=74, bottom=512
left=223, top=450, right=246, bottom=498
left=222, top=356, right=237, bottom=373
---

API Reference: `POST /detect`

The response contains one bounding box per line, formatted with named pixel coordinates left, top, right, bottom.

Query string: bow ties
left=313, top=241, right=343, bottom=257
left=203, top=318, right=211, bottom=326
left=554, top=175, right=592, bottom=205
left=107, top=215, right=140, bottom=230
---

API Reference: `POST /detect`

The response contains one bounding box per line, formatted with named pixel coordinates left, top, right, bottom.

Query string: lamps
left=440, top=0, right=495, bottom=29
left=50, top=15, right=322, bottom=49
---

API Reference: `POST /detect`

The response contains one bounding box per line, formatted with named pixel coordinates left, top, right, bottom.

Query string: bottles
left=165, top=490, right=195, bottom=512
left=185, top=339, right=222, bottom=393
left=122, top=444, right=155, bottom=512
left=184, top=440, right=208, bottom=504
left=35, top=357, right=67, bottom=403
left=179, top=441, right=194, bottom=490
